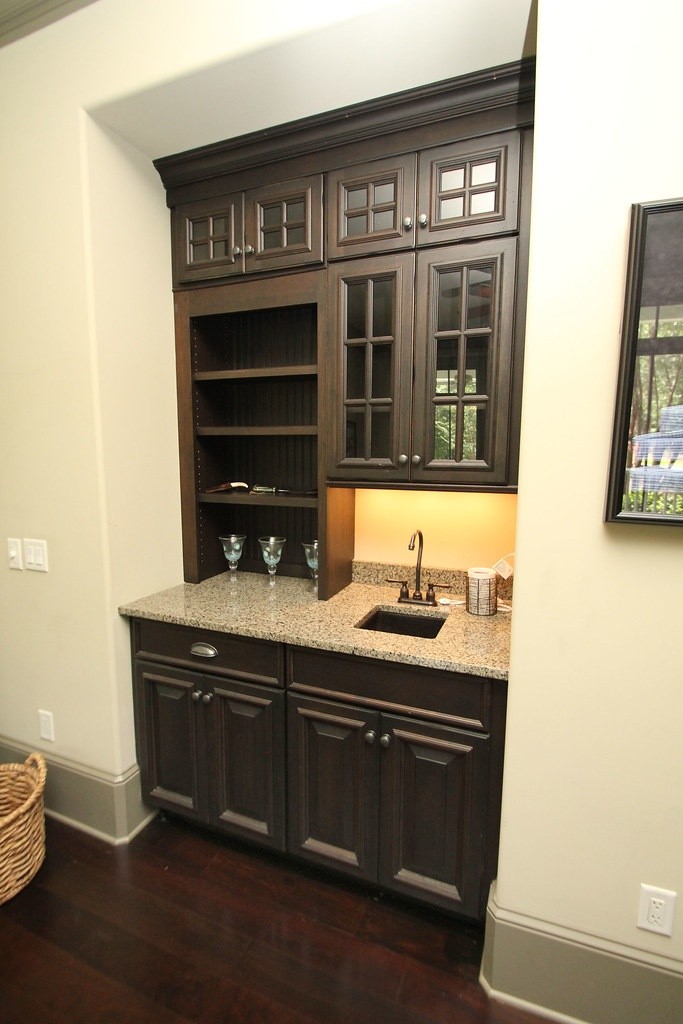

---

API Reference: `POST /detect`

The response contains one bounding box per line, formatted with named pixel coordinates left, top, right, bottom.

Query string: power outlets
left=38, top=709, right=54, bottom=742
left=636, top=883, right=677, bottom=937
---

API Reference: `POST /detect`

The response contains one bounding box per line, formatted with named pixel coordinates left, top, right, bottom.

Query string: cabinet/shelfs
left=324, top=130, right=519, bottom=263
left=285, top=644, right=508, bottom=928
left=328, top=236, right=520, bottom=495
left=128, top=616, right=286, bottom=857
left=170, top=174, right=324, bottom=289
left=173, top=268, right=355, bottom=602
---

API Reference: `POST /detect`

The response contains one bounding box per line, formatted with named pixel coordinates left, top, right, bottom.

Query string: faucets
left=407, top=529, right=424, bottom=600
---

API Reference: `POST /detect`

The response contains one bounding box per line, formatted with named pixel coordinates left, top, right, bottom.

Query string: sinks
left=353, top=605, right=450, bottom=639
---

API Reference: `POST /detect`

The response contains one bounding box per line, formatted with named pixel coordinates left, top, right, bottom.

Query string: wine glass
left=218, top=535, right=246, bottom=582
left=258, top=536, right=286, bottom=587
left=302, top=540, right=319, bottom=594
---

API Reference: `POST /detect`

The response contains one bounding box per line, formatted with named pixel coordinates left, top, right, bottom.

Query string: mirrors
left=601, top=198, right=683, bottom=525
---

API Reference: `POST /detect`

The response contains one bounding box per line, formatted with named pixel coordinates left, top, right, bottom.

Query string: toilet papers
left=467, top=567, right=497, bottom=615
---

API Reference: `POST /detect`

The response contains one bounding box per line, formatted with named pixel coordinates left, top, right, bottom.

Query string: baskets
left=0, top=751, right=48, bottom=905
